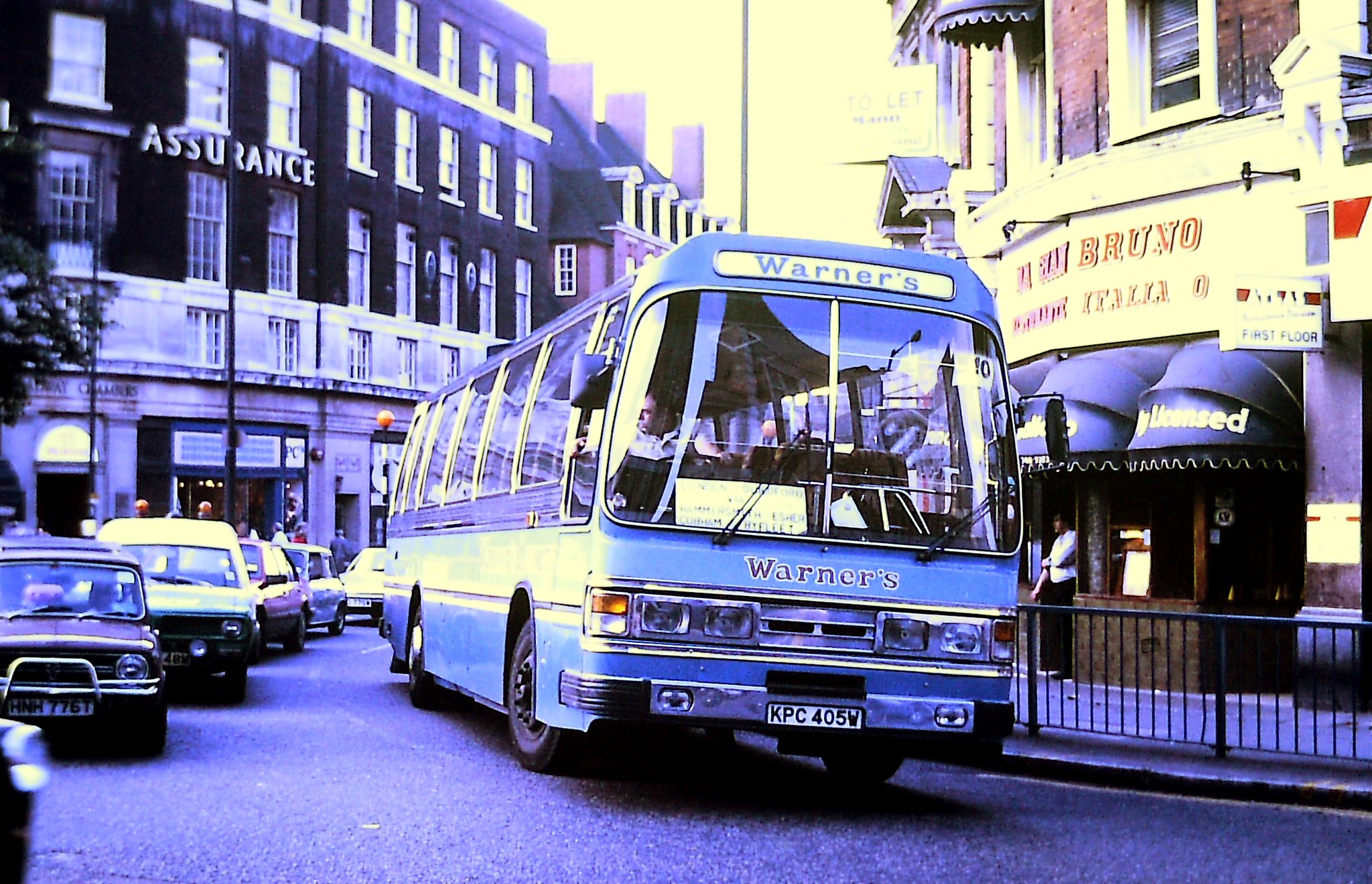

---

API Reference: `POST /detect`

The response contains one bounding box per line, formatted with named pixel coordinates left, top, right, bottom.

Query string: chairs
left=715, top=445, right=915, bottom=515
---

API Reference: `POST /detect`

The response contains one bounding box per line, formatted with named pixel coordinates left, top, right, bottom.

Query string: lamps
left=1240, top=162, right=1301, bottom=191
left=1002, top=217, right=1072, bottom=243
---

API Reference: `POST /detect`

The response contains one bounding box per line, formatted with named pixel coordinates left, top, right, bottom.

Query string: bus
left=383, top=231, right=1073, bottom=786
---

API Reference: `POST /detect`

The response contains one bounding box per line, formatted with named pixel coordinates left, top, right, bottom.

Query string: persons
left=330, top=529, right=351, bottom=575
left=574, top=385, right=734, bottom=467
left=293, top=522, right=309, bottom=544
left=1031, top=509, right=1076, bottom=680
left=235, top=522, right=248, bottom=537
left=271, top=522, right=289, bottom=542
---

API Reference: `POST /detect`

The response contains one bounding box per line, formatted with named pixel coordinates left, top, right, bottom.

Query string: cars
left=284, top=543, right=348, bottom=637
left=0, top=539, right=171, bottom=755
left=236, top=538, right=315, bottom=656
left=336, top=547, right=387, bottom=625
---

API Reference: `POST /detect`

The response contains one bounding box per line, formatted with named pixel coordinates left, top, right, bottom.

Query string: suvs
left=94, top=500, right=290, bottom=701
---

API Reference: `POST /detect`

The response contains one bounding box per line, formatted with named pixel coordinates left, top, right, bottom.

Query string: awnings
left=933, top=0, right=1044, bottom=53
left=922, top=337, right=1306, bottom=468
